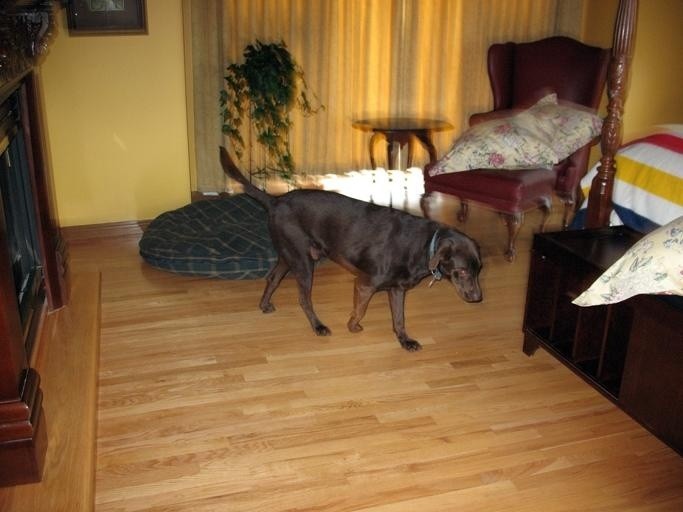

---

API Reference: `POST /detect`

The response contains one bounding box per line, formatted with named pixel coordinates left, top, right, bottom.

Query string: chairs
left=415, top=31, right=615, bottom=261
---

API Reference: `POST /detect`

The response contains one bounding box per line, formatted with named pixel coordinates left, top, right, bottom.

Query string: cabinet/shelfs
left=521, top=225, right=681, bottom=454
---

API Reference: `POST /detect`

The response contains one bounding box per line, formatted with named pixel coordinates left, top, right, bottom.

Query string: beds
left=576, top=121, right=683, bottom=229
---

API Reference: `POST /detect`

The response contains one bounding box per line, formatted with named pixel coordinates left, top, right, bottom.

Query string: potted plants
left=215, top=36, right=324, bottom=198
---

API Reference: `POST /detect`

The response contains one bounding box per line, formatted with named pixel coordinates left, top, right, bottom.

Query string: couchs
left=130, top=186, right=343, bottom=284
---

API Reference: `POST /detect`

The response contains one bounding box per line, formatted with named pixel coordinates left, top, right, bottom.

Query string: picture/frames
left=62, top=0, right=152, bottom=39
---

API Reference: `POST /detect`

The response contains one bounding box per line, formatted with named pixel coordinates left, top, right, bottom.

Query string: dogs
left=218, top=145, right=484, bottom=352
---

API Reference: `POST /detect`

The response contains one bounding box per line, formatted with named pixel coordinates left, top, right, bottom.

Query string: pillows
left=567, top=214, right=683, bottom=307
left=422, top=89, right=606, bottom=182
